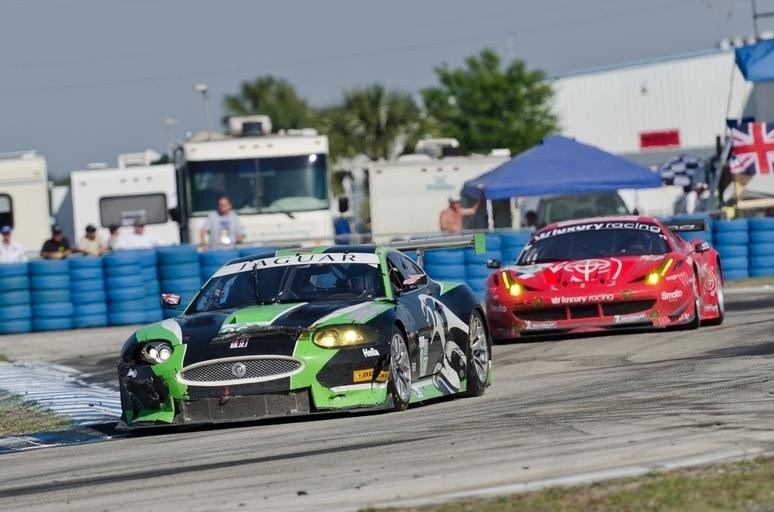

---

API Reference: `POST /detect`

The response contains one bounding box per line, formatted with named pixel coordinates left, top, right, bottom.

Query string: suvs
left=520, top=191, right=640, bottom=230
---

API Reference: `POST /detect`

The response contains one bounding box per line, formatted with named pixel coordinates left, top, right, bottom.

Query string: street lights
left=192, top=82, right=216, bottom=142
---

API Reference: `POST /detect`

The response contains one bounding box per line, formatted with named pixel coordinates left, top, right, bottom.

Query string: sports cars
left=112, top=248, right=494, bottom=429
left=482, top=216, right=726, bottom=341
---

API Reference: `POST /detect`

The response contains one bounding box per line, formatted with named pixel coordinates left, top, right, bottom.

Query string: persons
left=671, top=181, right=707, bottom=213
left=39, top=217, right=149, bottom=259
left=0, top=224, right=30, bottom=264
left=345, top=263, right=378, bottom=300
left=333, top=196, right=372, bottom=245
left=521, top=206, right=545, bottom=231
left=439, top=184, right=485, bottom=236
left=200, top=196, right=247, bottom=252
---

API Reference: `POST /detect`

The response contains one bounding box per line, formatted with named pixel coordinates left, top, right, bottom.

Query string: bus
left=166, top=113, right=335, bottom=250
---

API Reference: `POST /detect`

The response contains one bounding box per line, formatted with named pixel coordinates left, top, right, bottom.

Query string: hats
left=51, top=224, right=63, bottom=235
left=85, top=225, right=96, bottom=234
left=133, top=218, right=148, bottom=228
left=110, top=223, right=121, bottom=233
left=449, top=193, right=462, bottom=204
left=0, top=225, right=13, bottom=235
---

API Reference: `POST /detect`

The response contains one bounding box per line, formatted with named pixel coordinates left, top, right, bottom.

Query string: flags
left=731, top=120, right=774, bottom=174
